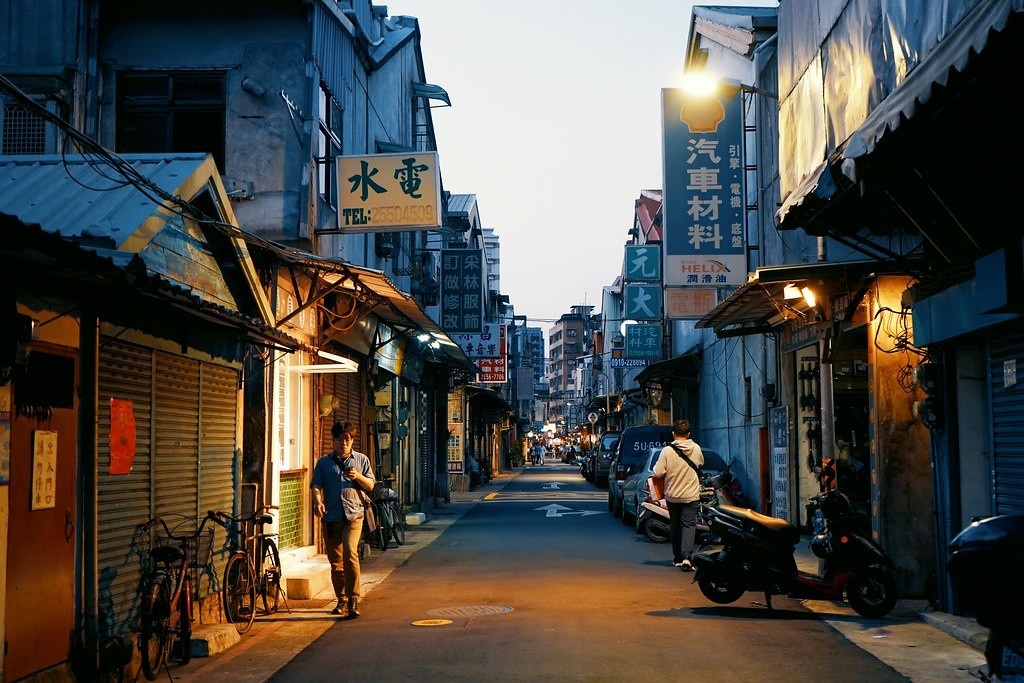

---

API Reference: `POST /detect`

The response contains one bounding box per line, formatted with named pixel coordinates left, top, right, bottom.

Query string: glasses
left=335, top=438, right=353, bottom=445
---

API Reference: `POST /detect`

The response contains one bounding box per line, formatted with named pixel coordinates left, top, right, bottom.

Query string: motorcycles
left=690, top=457, right=901, bottom=619
left=636, top=455, right=758, bottom=544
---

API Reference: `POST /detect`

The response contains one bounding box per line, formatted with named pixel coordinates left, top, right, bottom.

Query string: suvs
left=607, top=425, right=696, bottom=520
left=594, top=431, right=621, bottom=488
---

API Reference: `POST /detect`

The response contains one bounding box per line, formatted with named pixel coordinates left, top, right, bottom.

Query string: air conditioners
left=421, top=251, right=436, bottom=281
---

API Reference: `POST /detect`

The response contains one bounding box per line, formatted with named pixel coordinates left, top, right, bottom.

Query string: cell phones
left=345, top=466, right=351, bottom=475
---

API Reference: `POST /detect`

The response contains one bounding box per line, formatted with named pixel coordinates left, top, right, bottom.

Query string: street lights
left=682, top=67, right=836, bottom=492
left=579, top=368, right=610, bottom=431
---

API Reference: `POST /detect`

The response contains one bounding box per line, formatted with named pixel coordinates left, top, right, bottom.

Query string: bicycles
left=365, top=478, right=407, bottom=551
left=216, top=504, right=292, bottom=634
left=127, top=510, right=226, bottom=683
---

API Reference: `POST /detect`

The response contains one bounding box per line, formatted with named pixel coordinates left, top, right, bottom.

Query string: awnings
left=519, top=417, right=530, bottom=424
left=693, top=278, right=875, bottom=336
left=773, top=0, right=1024, bottom=230
left=300, top=258, right=484, bottom=381
left=589, top=393, right=619, bottom=409
left=466, top=384, right=512, bottom=414
left=633, top=352, right=702, bottom=379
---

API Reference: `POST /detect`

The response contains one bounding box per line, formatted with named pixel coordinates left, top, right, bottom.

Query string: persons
left=527, top=427, right=600, bottom=478
left=652, top=418, right=704, bottom=571
left=467, top=451, right=485, bottom=492
left=310, top=421, right=376, bottom=617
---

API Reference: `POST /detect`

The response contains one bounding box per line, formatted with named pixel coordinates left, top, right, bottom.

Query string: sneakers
left=347, top=598, right=360, bottom=618
left=682, top=556, right=696, bottom=571
left=672, top=556, right=682, bottom=567
left=332, top=600, right=348, bottom=615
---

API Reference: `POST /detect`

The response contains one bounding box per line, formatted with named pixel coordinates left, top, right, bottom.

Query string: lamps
left=781, top=284, right=824, bottom=321
left=379, top=233, right=394, bottom=263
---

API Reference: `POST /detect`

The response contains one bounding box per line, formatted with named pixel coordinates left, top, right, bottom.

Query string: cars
left=620, top=443, right=747, bottom=532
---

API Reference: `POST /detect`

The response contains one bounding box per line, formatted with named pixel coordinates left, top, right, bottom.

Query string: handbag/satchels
left=364, top=493, right=379, bottom=534
left=646, top=476, right=665, bottom=501
